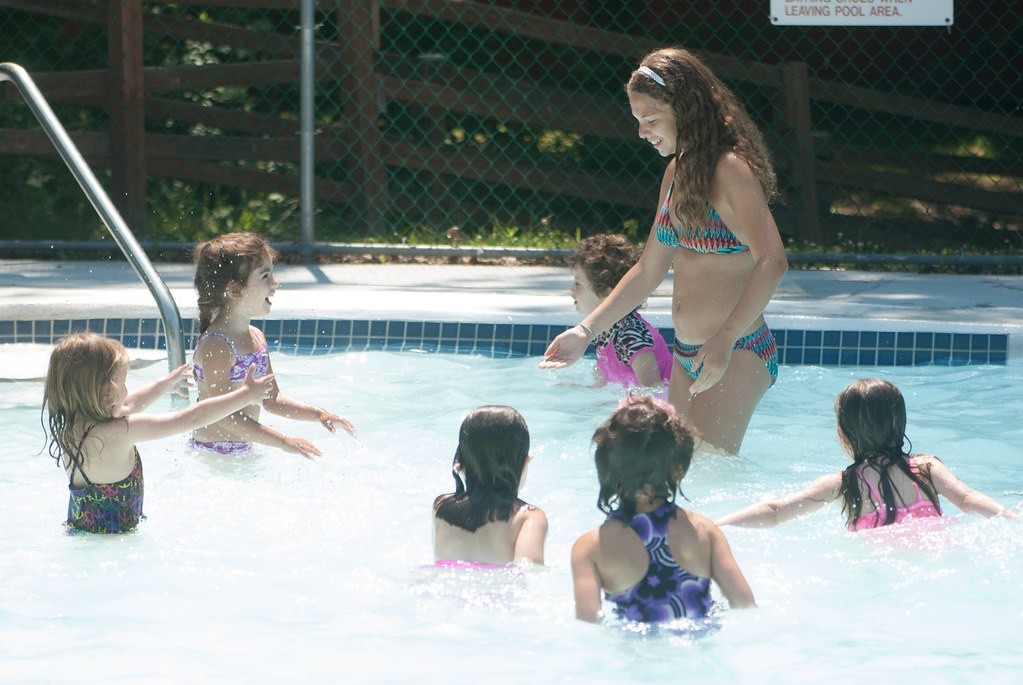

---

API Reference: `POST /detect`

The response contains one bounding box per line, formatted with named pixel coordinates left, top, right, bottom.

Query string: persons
left=431, top=403, right=548, bottom=568
left=571, top=394, right=753, bottom=625
left=715, top=378, right=1017, bottom=533
left=35, top=333, right=273, bottom=536
left=537, top=49, right=788, bottom=460
left=570, top=235, right=673, bottom=387
left=186, top=231, right=357, bottom=459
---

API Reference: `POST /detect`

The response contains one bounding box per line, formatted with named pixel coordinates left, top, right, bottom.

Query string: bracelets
left=577, top=322, right=595, bottom=343
